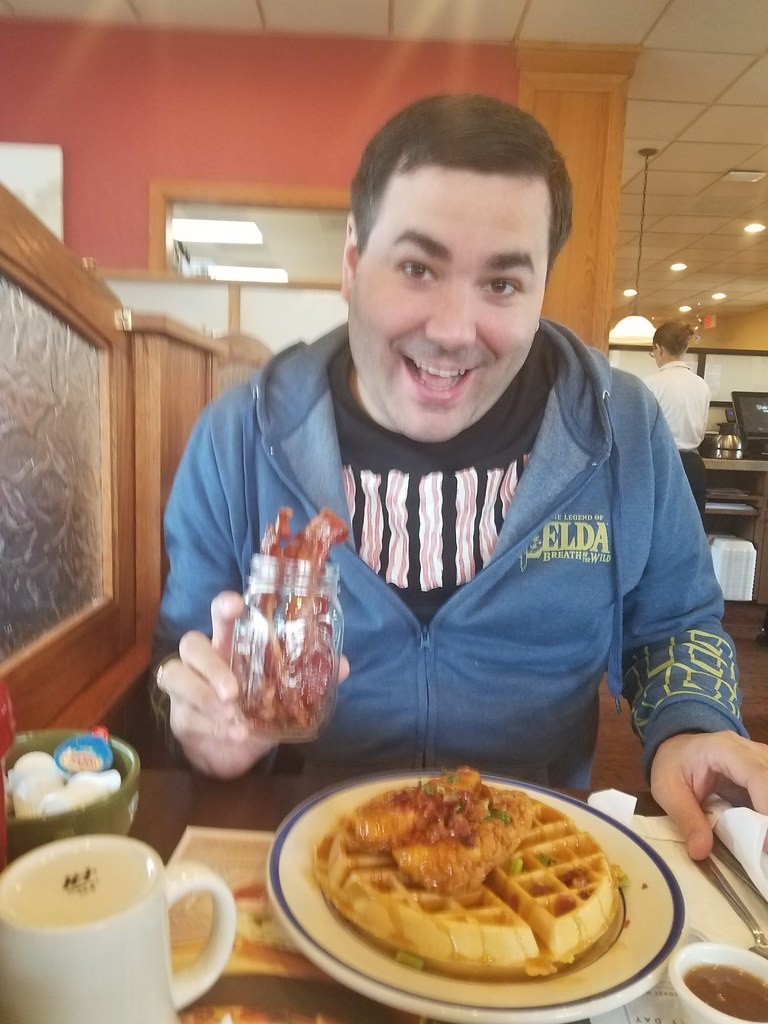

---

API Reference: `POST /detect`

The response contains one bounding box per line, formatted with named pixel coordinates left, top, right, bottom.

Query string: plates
left=264, top=771, right=691, bottom=1024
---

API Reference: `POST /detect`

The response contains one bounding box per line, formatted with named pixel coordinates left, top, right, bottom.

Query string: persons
left=145, top=94, right=768, bottom=863
left=641, top=320, right=710, bottom=524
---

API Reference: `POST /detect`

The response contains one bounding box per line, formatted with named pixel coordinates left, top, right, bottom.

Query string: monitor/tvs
left=732, top=391, right=768, bottom=460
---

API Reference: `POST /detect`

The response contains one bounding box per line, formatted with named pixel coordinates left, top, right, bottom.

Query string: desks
left=700, top=456, right=768, bottom=603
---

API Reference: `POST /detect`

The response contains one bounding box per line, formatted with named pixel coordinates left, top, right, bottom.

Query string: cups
left=0, top=834, right=236, bottom=1024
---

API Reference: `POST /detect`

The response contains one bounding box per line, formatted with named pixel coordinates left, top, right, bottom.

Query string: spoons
left=688, top=853, right=768, bottom=960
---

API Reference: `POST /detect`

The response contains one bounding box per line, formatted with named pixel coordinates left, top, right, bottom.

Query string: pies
left=325, top=773, right=625, bottom=981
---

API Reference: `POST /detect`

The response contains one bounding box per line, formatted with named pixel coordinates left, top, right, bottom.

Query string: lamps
left=606, top=148, right=658, bottom=346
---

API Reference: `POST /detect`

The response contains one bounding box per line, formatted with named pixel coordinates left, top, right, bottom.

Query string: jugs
left=710, top=421, right=749, bottom=460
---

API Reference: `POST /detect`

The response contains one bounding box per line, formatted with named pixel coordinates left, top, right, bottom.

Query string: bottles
left=230, top=557, right=346, bottom=744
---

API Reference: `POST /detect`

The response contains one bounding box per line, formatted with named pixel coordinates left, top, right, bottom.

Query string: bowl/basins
left=3, top=729, right=142, bottom=865
left=669, top=940, right=768, bottom=1024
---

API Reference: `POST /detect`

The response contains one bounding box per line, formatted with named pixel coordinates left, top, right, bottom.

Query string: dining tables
left=128, top=769, right=668, bottom=1024
